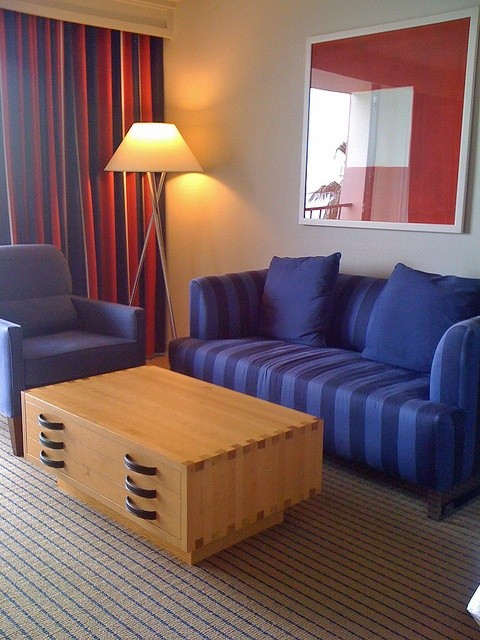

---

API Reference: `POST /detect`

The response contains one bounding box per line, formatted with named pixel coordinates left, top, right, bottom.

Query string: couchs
left=0, top=244, right=146, bottom=457
left=168, top=251, right=479, bottom=523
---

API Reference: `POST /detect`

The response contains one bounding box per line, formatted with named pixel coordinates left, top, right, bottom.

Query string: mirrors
left=297, top=9, right=478, bottom=233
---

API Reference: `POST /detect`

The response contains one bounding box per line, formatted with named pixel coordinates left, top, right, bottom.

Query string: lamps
left=102, top=121, right=206, bottom=340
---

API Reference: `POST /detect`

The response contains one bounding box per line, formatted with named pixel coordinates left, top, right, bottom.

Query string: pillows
left=360, top=263, right=480, bottom=376
left=257, top=252, right=341, bottom=348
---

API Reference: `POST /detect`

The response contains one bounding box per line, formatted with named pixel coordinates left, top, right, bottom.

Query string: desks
left=21, top=362, right=324, bottom=566
